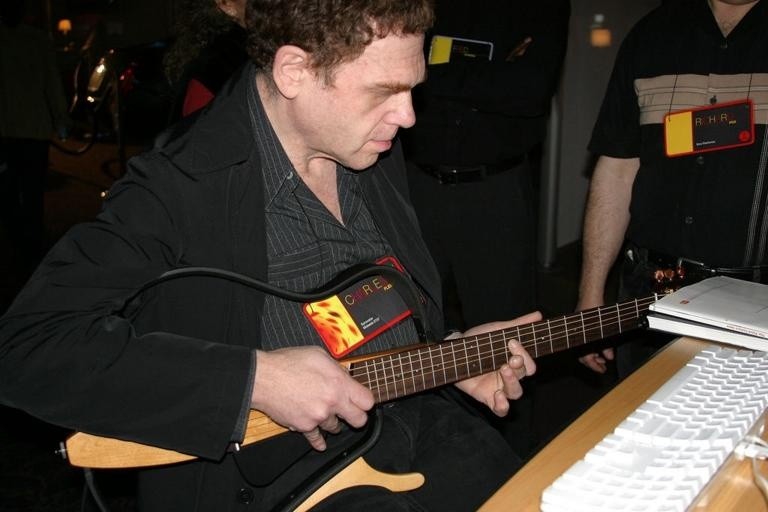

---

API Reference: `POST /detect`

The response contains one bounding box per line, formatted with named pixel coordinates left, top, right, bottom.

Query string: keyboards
left=540, top=344, right=768, bottom=512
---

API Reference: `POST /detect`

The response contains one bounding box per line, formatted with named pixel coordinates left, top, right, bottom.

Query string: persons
left=0, top=0, right=545, bottom=512
left=570, top=1, right=768, bottom=383
left=179, top=0, right=251, bottom=120
left=395, top=0, right=571, bottom=461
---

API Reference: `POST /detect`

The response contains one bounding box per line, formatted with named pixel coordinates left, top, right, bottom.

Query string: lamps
left=592, top=29, right=611, bottom=46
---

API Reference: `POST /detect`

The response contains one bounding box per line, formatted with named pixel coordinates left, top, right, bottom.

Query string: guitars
left=55, top=258, right=740, bottom=511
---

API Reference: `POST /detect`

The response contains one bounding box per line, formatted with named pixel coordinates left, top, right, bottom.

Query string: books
left=428, top=34, right=494, bottom=67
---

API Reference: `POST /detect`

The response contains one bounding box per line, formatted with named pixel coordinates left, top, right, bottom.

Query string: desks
left=473, top=335, right=768, bottom=512
